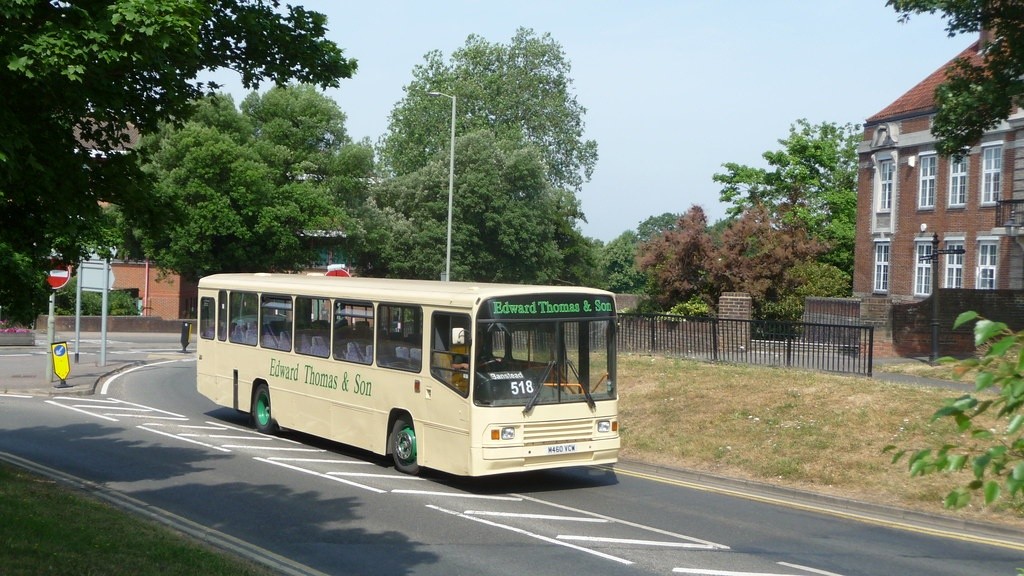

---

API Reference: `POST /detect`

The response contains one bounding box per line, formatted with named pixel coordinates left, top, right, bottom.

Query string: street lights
left=429, top=89, right=458, bottom=281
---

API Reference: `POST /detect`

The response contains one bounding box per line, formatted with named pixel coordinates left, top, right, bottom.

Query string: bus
left=195, top=270, right=622, bottom=480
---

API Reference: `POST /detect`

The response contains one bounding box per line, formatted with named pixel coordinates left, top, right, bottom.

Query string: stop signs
left=38, top=257, right=73, bottom=288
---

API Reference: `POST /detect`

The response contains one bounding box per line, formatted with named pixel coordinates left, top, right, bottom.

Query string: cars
left=230, top=313, right=285, bottom=339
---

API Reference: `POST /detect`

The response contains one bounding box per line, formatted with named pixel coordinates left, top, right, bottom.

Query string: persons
left=452, top=331, right=502, bottom=370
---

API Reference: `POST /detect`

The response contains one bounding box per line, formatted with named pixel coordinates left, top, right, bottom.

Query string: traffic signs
left=937, top=249, right=966, bottom=254
left=919, top=254, right=934, bottom=261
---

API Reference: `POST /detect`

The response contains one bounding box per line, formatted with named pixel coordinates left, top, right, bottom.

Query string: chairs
left=204, top=320, right=423, bottom=365
